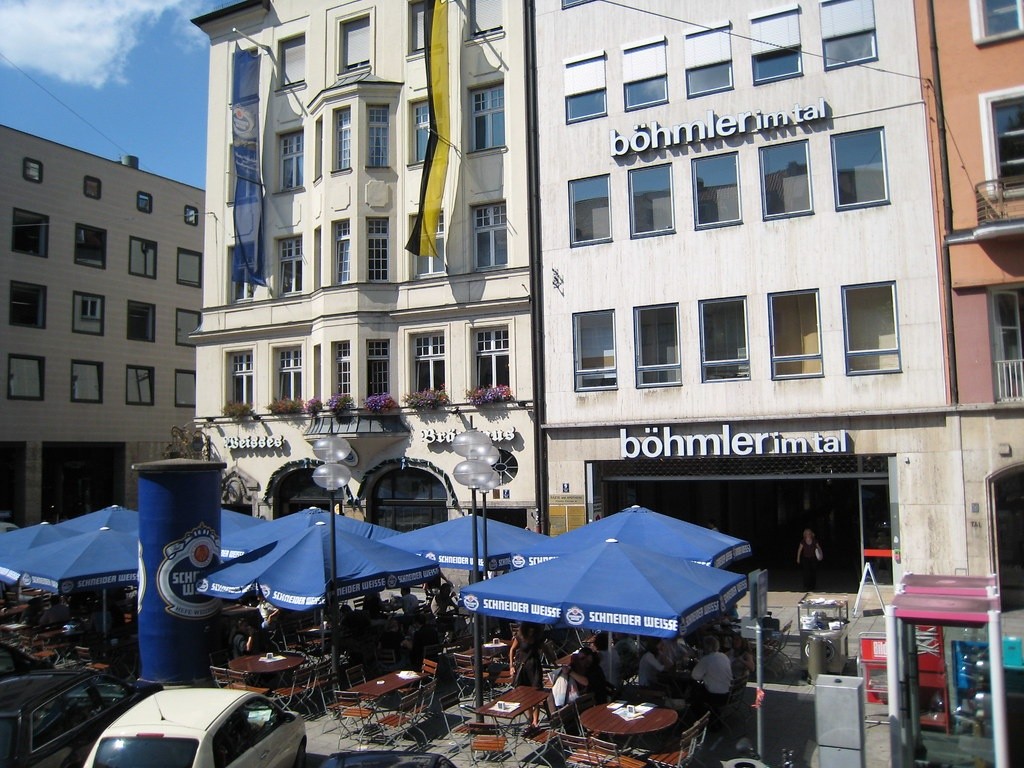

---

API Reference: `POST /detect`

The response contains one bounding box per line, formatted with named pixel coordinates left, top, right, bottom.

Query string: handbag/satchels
left=814, top=539, right=822, bottom=561
left=511, top=664, right=524, bottom=688
left=548, top=665, right=580, bottom=706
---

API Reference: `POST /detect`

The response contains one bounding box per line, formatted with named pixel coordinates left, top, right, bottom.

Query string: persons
left=706, top=519, right=720, bottom=532
left=230, top=569, right=510, bottom=686
left=550, top=601, right=755, bottom=737
left=509, top=620, right=557, bottom=732
left=796, top=529, right=824, bottom=590
left=20, top=587, right=138, bottom=642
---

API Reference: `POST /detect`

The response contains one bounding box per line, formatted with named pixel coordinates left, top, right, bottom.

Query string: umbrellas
left=198, top=522, right=440, bottom=651
left=218, top=506, right=405, bottom=562
left=460, top=537, right=747, bottom=701
left=509, top=504, right=752, bottom=570
left=380, top=514, right=551, bottom=581
left=0, top=503, right=266, bottom=634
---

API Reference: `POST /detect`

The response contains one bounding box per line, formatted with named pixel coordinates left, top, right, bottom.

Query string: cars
left=0, top=666, right=166, bottom=768
left=81, top=687, right=307, bottom=768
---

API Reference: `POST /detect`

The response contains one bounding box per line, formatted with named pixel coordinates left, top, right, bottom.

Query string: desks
left=665, top=655, right=704, bottom=715
left=477, top=685, right=551, bottom=743
left=37, top=625, right=86, bottom=662
left=298, top=622, right=345, bottom=656
left=456, top=636, right=514, bottom=700
left=217, top=604, right=263, bottom=643
left=89, top=632, right=138, bottom=671
left=2, top=600, right=41, bottom=618
left=579, top=700, right=678, bottom=765
left=0, top=618, right=36, bottom=653
left=228, top=648, right=305, bottom=703
left=346, top=668, right=428, bottom=743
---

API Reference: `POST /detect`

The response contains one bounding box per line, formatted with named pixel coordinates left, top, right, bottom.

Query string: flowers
left=215, top=384, right=512, bottom=419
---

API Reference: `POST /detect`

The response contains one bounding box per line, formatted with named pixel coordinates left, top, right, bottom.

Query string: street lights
left=312, top=433, right=352, bottom=701
left=452, top=414, right=493, bottom=724
left=478, top=434, right=501, bottom=644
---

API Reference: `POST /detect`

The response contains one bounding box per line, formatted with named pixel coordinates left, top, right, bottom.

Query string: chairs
left=0, top=558, right=801, bottom=768
